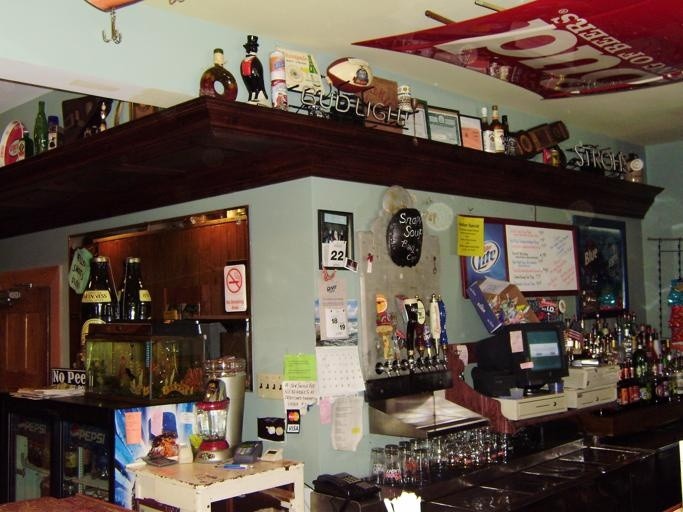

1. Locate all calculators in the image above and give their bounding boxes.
[142,455,177,467]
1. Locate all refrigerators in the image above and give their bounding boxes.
[8,391,202,512]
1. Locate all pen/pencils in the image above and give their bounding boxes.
[224,464,248,467]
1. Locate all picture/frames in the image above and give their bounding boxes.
[319,209,355,270]
[460,212,581,299]
[404,99,484,151]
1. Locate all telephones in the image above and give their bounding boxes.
[313,472,382,500]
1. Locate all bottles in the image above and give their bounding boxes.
[566,311,683,406]
[308,55,321,87]
[27,437,109,500]
[117,257,151,321]
[502,114,516,156]
[78,256,117,369]
[18,128,33,162]
[33,101,49,156]
[489,106,505,155]
[481,107,495,154]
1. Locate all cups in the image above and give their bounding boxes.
[368,427,511,486]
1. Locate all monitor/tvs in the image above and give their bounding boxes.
[490,321,569,398]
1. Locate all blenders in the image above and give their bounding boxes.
[195,400,231,465]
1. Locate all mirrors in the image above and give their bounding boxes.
[572,215,630,320]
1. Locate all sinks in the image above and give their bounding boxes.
[529,458,601,478]
[483,470,572,495]
[564,446,640,468]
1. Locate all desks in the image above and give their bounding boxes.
[134,458,308,512]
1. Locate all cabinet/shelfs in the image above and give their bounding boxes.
[449,344,683,456]
[0,205,252,512]
